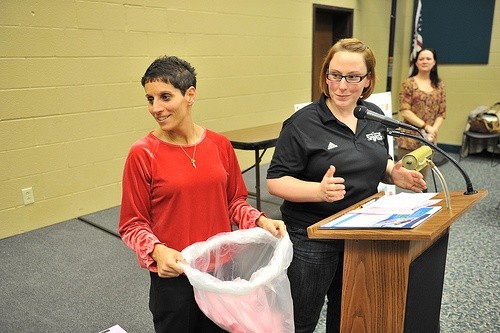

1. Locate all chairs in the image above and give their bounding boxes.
[463,113,500,167]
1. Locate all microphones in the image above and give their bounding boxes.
[353,105,418,131]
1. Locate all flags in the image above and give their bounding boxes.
[409,0,423,77]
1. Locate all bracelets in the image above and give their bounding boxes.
[423,122,427,128]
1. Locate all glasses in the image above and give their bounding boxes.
[326,72,369,83]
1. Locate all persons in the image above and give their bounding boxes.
[117,56,286,333]
[266,38,427,333]
[397,47,447,193]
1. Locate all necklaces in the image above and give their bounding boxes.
[164,123,196,168]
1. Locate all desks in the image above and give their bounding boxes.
[220,123,284,212]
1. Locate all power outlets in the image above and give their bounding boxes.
[22,186,35,205]
